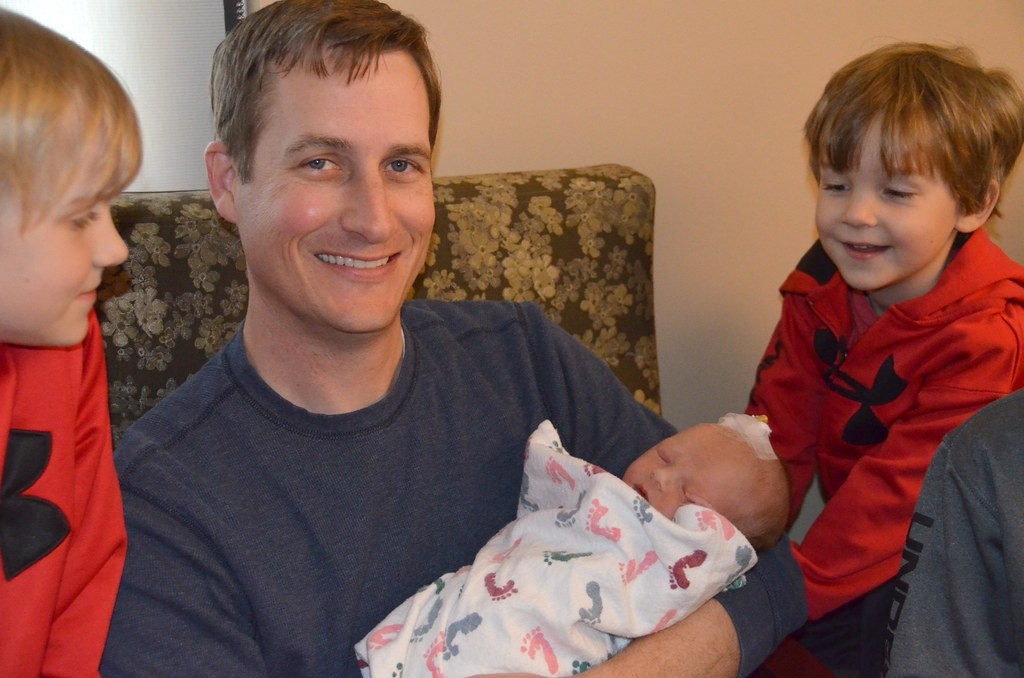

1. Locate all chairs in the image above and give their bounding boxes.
[91,161,665,449]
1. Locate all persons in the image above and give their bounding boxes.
[100,0,807,677]
[882,390,1024,677]
[0,4,144,677]
[741,38,1024,677]
[355,414,790,678]
